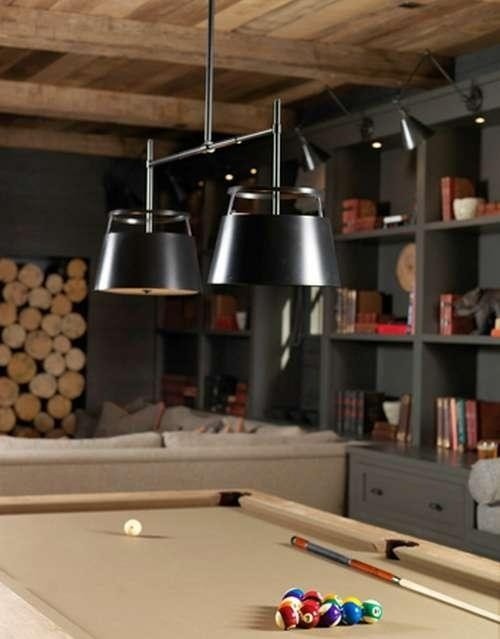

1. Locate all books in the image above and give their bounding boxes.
[334,389,413,442]
[440,293,476,335]
[209,293,246,332]
[342,197,380,233]
[163,371,247,416]
[436,396,500,453]
[441,174,475,221]
[334,286,382,334]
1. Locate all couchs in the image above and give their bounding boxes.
[0,404,348,518]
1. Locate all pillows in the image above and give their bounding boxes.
[73,396,245,439]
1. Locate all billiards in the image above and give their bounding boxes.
[124,520,141,536]
[275,588,383,630]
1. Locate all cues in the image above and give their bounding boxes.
[291,535,499,622]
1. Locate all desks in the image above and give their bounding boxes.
[0,487,500,639]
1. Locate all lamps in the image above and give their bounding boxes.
[391,48,484,154]
[93,1,343,297]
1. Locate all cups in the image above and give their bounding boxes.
[475,438,500,462]
